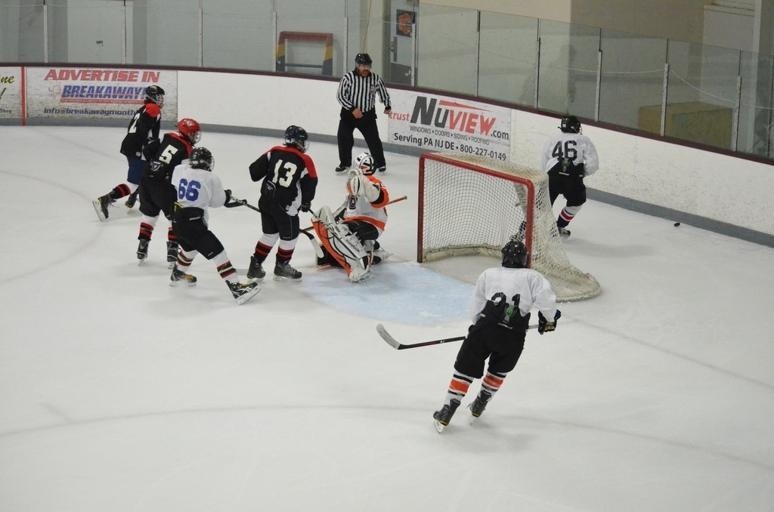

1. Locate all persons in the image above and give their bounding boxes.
[90,84,166,218]
[432,240,553,426]
[133,118,199,265]
[335,52,392,173]
[512,115,599,241]
[316,153,388,270]
[248,125,319,281]
[170,147,259,304]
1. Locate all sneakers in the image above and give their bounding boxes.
[433,398,461,426]
[136,243,148,259]
[166,250,180,262]
[273,254,303,279]
[98,194,117,219]
[469,389,492,417]
[170,263,197,283]
[379,163,387,171]
[246,256,266,279]
[226,279,257,299]
[335,162,348,171]
[549,227,571,237]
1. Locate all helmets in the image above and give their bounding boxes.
[189,146,215,172]
[174,117,201,146]
[353,152,378,175]
[283,125,310,153]
[559,113,582,134]
[143,84,165,109]
[355,53,373,64]
[501,240,529,269]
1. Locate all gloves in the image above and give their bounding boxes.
[538,309,562,335]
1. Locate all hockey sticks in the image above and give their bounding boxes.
[228,194,325,259]
[375,323,540,350]
[224,199,247,208]
